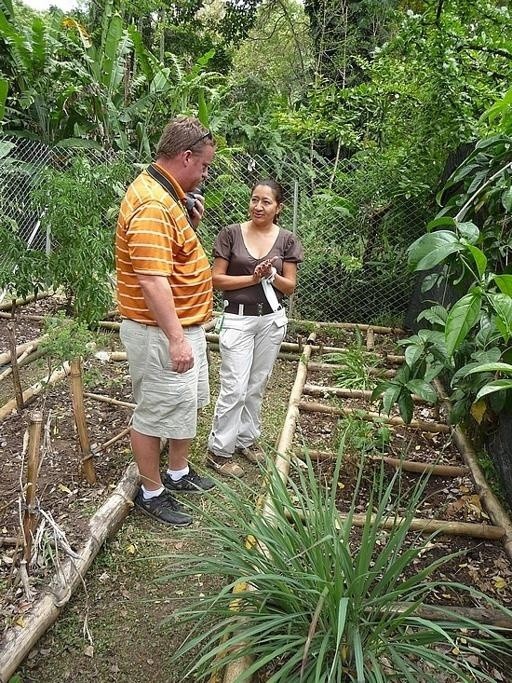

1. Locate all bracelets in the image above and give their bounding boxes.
[264,267,279,284]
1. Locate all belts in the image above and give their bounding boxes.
[225,299,282,316]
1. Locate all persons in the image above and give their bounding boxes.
[113,115,220,527]
[205,177,303,479]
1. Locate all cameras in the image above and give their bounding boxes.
[185,185,205,219]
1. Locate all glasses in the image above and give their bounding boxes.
[186,129,213,151]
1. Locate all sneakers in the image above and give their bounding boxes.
[133,487,193,526]
[163,464,216,493]
[239,443,266,464]
[205,449,245,478]
